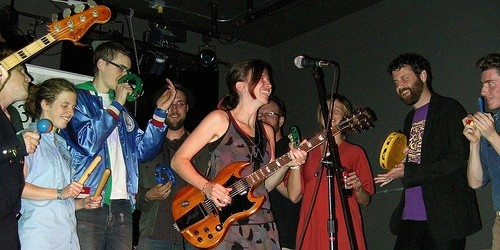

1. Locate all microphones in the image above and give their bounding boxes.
[294,55,330,68]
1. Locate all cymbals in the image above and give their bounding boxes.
[382,131,407,169]
[118,70,145,102]
[292,126,299,146]
[153,163,176,186]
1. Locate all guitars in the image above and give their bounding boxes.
[0,0,112,76]
[170,106,379,249]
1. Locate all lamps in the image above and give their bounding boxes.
[131,18,179,96]
[195,30,219,71]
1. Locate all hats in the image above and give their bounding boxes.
[153,63,196,113]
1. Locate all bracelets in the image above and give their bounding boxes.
[290,166,301,170]
[203,183,207,192]
[81,200,84,208]
[57,189,62,200]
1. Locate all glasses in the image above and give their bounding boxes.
[258,111,281,118]
[169,101,187,109]
[103,58,131,75]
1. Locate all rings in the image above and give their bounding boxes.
[389,178,391,180]
[164,190,168,192]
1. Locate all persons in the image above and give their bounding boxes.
[283,93,375,250]
[170,60,306,250]
[256,96,302,250]
[0,42,210,250]
[462,53,500,250]
[372,52,483,250]
